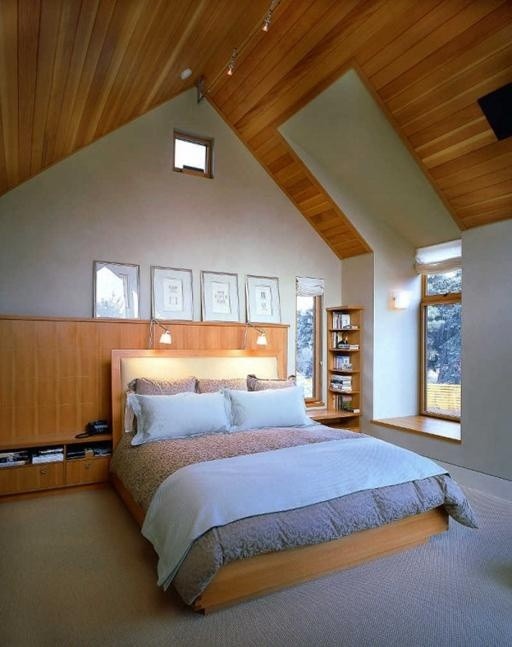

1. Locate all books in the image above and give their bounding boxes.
[330,311,360,413]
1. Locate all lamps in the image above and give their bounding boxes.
[238,323,269,352]
[142,318,174,351]
[196,0,280,105]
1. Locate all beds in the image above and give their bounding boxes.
[109,348,482,614]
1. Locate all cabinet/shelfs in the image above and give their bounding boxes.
[326,303,365,437]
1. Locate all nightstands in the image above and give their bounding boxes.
[305,407,362,433]
[0,432,112,499]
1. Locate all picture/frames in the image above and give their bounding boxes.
[148,264,195,323]
[198,268,241,323]
[246,270,280,323]
[90,260,141,321]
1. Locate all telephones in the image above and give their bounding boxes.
[87,419,110,436]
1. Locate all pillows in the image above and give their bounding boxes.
[127,376,197,434]
[250,373,296,391]
[128,391,231,445]
[222,388,311,433]
[191,376,247,392]
